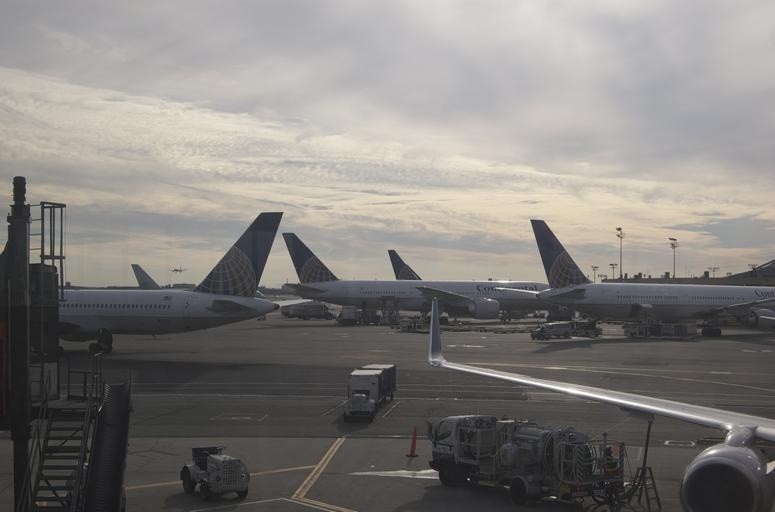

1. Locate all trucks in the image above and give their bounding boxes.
[425,414,627,509]
[180,444,251,500]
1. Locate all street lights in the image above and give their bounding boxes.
[591,225,678,280]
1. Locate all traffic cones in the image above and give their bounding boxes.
[405,426,419,457]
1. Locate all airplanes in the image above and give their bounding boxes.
[281,231,549,322]
[57,210,284,355]
[530,218,775,338]
